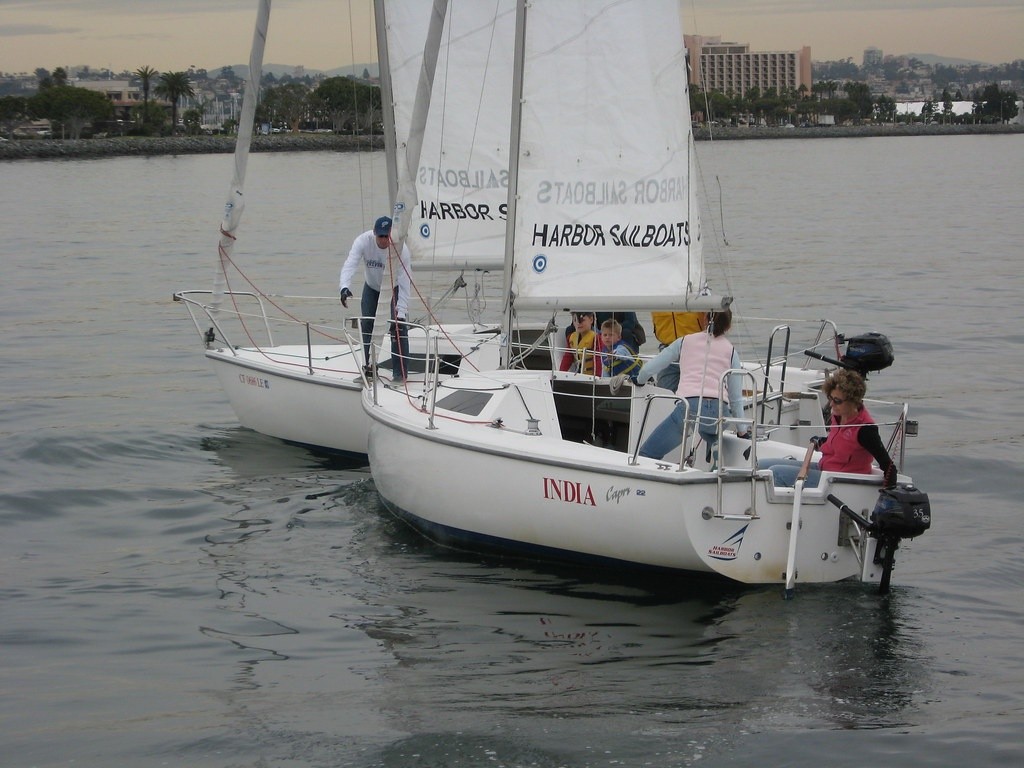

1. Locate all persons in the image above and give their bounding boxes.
[650,311,707,393]
[560,312,656,382]
[756,369,898,488]
[629,308,749,460]
[340,215,412,386]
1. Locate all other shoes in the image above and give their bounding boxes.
[353,371,378,383]
[388,378,406,387]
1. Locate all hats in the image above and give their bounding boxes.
[374,216,392,235]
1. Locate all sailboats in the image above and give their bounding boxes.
[168,0,895,460]
[336,0,933,589]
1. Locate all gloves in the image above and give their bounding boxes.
[632,376,645,387]
[737,433,749,439]
[341,288,353,308]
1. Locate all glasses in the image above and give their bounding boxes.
[379,234,388,239]
[827,395,847,405]
